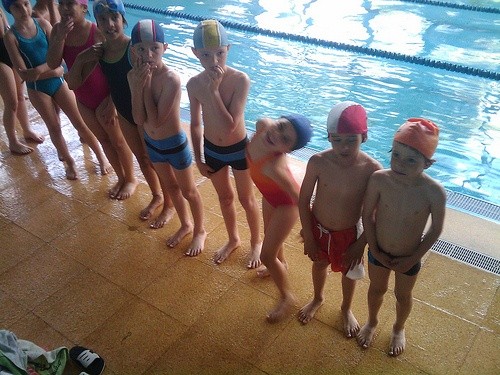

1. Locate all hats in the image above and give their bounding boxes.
[393,116,439,160]
[193,19,231,52]
[92,0,126,20]
[130,18,165,46]
[326,100,369,135]
[281,113,314,151]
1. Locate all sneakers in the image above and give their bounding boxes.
[69,345,106,375]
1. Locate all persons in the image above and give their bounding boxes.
[185,19,263,269]
[354,117,447,356]
[0,0,208,257]
[298,100,384,338]
[245,113,312,324]
